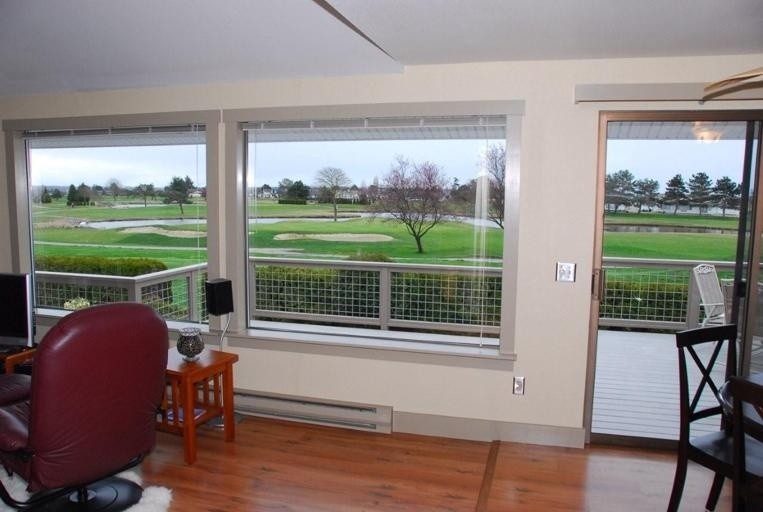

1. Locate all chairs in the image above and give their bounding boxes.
[666,323,763,511]
[692,264,726,327]
[0,302,168,511]
[732,378,763,512]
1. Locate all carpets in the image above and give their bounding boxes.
[0,464,172,511]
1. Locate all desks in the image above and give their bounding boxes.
[717,373,763,511]
[154,344,238,465]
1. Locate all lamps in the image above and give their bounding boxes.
[204,277,241,427]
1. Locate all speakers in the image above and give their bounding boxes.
[205,279,233,315]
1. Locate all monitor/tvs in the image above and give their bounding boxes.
[0,272,33,353]
